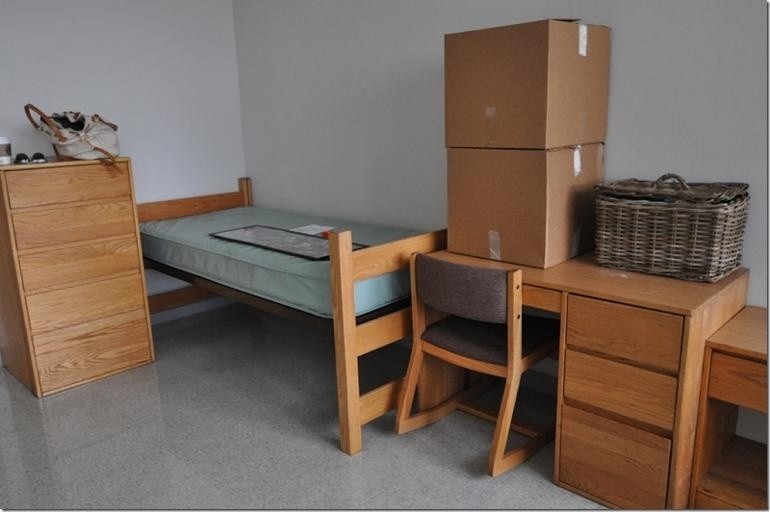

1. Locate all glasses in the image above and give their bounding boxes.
[15,153,46,163]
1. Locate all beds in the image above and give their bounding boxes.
[137,178,447,456]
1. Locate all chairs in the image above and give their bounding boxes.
[395,253,555,477]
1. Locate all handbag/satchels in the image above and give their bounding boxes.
[24,104,119,160]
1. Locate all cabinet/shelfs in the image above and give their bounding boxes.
[688,306,768,510]
[416,249,749,510]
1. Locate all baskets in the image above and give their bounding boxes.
[593,177,750,283]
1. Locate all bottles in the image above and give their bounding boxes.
[0,137,12,165]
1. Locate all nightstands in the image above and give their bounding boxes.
[0,157,155,399]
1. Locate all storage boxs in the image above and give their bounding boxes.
[444,18,611,150]
[447,143,606,269]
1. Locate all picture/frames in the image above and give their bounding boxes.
[208,224,370,260]
